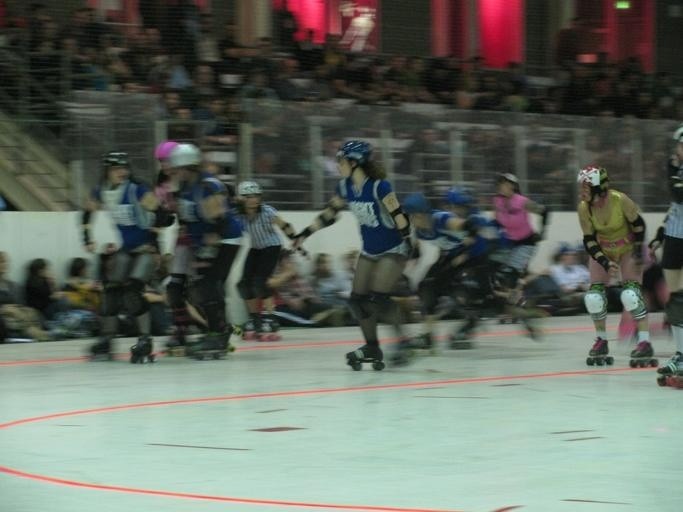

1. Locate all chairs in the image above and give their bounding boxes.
[74,131,682,213]
[6,37,683,131]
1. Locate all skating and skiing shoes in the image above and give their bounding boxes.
[165,328,236,359]
[586,339,615,366]
[657,348,683,390]
[392,301,547,364]
[255,314,282,342]
[342,342,386,372]
[239,313,259,341]
[87,340,114,363]
[129,335,157,365]
[629,340,658,369]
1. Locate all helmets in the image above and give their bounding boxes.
[443,189,469,207]
[498,172,520,189]
[403,193,432,216]
[166,143,204,167]
[673,126,682,143]
[154,140,179,162]
[575,164,611,193]
[236,182,263,197]
[336,139,374,165]
[99,148,133,170]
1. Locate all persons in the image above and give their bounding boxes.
[491,171,549,317]
[439,184,529,339]
[291,139,422,364]
[148,139,199,346]
[404,194,541,351]
[77,149,156,358]
[576,166,656,358]
[162,145,243,357]
[235,179,310,331]
[646,126,682,373]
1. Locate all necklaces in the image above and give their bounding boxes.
[353,175,369,194]
